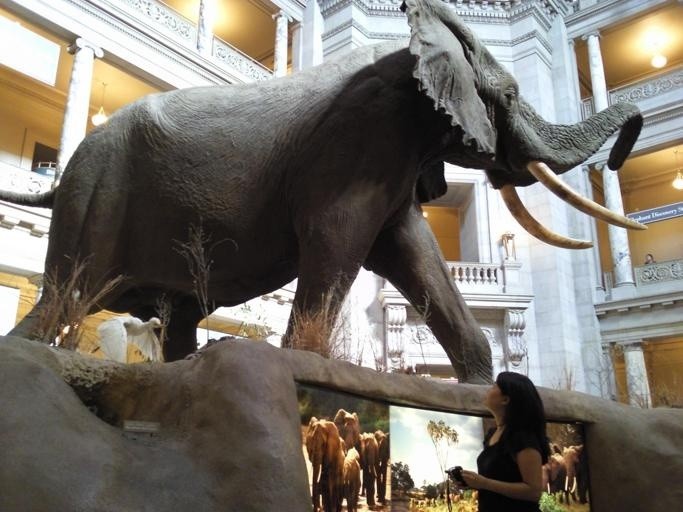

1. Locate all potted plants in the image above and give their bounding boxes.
[391,462,414,512]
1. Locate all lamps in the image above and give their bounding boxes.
[650,48,668,69]
[92,83,109,126]
[673,150,683,189]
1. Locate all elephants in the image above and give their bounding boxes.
[541,443,588,504]
[0,0,647,385]
[305,409,389,512]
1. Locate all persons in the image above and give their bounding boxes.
[642,253,660,282]
[445,370,549,512]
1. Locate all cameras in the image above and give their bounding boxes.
[449,467,464,481]
